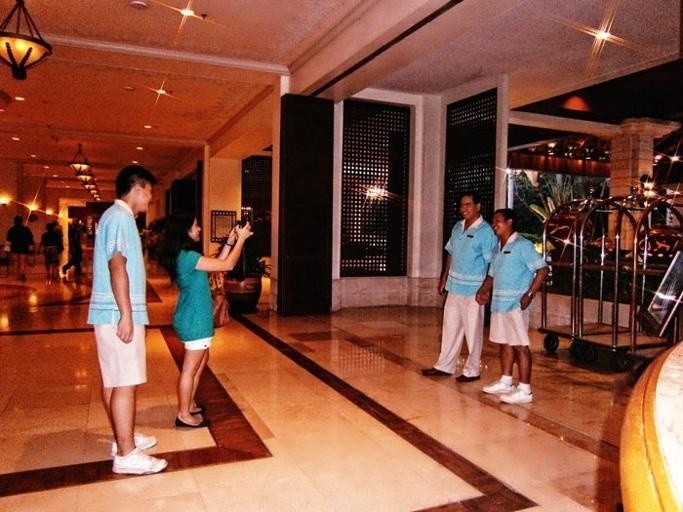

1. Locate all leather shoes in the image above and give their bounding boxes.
[175,417,209,427]
[423,369,451,376]
[190,406,204,414]
[456,375,480,382]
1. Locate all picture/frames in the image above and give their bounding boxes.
[210,210,237,243]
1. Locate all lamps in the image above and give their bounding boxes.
[0,0,52,80]
[69,142,103,202]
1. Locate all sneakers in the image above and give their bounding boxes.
[500,389,533,403]
[112,448,168,474]
[482,382,512,393]
[111,433,156,457]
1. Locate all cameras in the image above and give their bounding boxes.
[238,216,249,227]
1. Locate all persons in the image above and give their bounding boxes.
[85,165,168,475]
[62,217,84,276]
[475,209,550,406]
[161,211,255,427]
[37,220,65,280]
[6,216,35,281]
[421,190,498,382]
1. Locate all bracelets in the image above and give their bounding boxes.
[525,291,534,300]
[224,242,234,246]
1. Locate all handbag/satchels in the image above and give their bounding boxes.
[212,288,230,326]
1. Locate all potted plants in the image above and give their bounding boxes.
[215,214,272,314]
